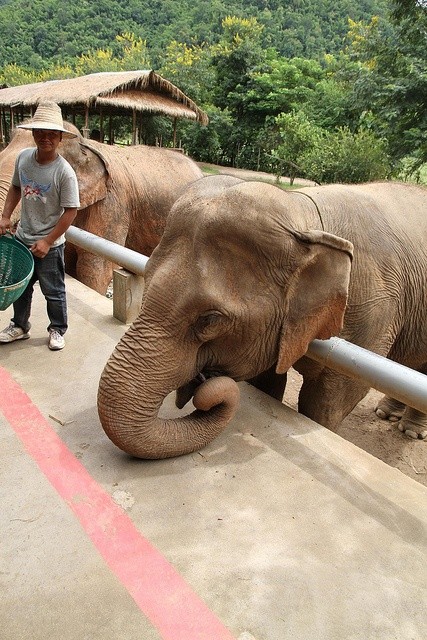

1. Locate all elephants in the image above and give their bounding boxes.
[98,171,427,460]
[0,120,203,297]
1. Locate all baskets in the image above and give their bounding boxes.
[1,229,35,311]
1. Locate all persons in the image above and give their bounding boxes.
[0,96,80,350]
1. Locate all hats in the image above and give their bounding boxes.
[17,100,78,139]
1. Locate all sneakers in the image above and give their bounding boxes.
[1,321,31,343]
[49,329,66,351]
